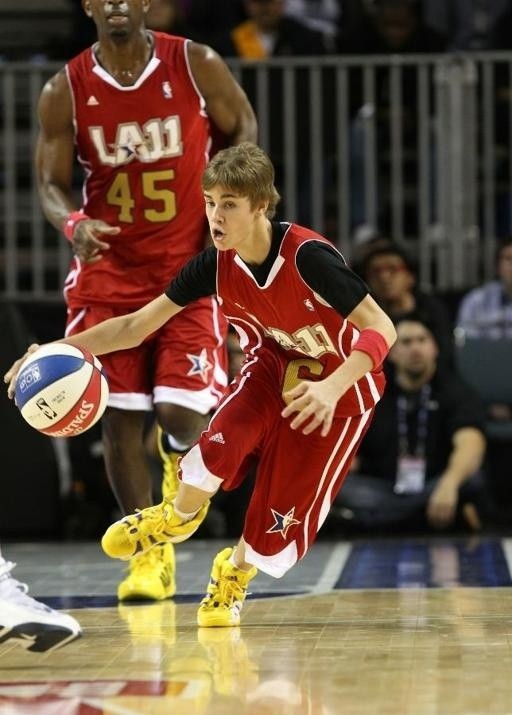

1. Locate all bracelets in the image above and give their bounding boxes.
[351,328,390,371]
[64,212,89,241]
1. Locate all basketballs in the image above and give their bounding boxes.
[14,342,109,438]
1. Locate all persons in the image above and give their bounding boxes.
[0,142,397,627]
[34,0,259,601]
[335,240,512,534]
[0,549,83,662]
[0,0,460,57]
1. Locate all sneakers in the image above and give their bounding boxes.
[0,555,84,655]
[102,425,211,602]
[195,546,258,627]
